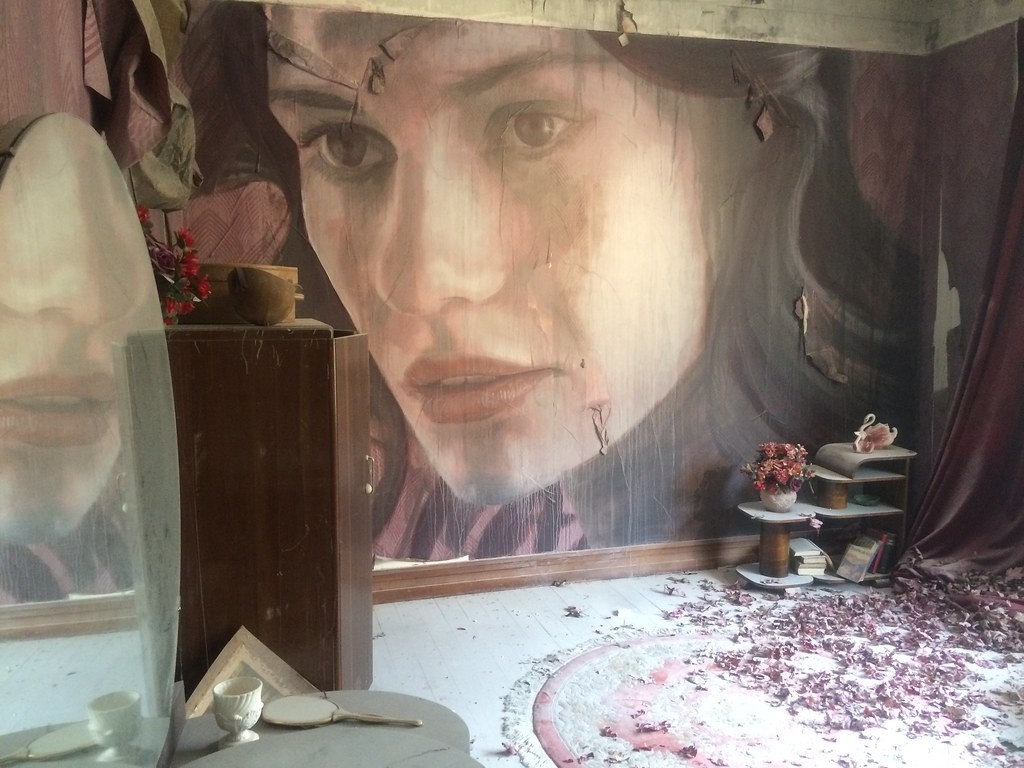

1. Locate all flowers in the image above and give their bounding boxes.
[737,441,816,495]
[136,205,212,326]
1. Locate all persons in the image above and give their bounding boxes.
[0,118,165,605]
[183,0,914,547]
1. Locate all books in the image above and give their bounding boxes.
[790,538,835,576]
[836,527,897,582]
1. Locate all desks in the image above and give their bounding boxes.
[0,690,485,768]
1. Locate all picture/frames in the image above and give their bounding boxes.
[186,625,322,718]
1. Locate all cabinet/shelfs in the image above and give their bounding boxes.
[735,501,817,590]
[797,444,916,584]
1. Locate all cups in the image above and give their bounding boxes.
[86,691,144,764]
[213,676,264,749]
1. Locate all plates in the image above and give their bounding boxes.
[853,494,881,505]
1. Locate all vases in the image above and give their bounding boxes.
[758,487,796,513]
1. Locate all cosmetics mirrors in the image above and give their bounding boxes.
[1,722,101,765]
[262,696,423,728]
